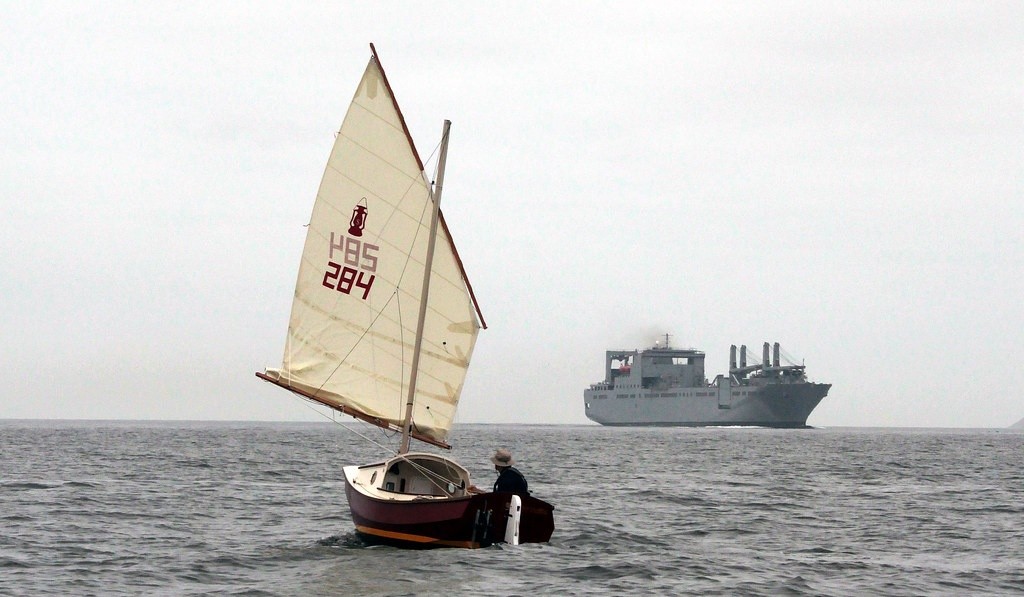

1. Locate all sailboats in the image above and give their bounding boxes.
[255,43,556,550]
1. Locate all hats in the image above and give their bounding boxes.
[491,448,514,467]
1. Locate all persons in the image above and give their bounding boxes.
[489,449,531,497]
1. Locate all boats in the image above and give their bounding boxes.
[583,333,832,428]
